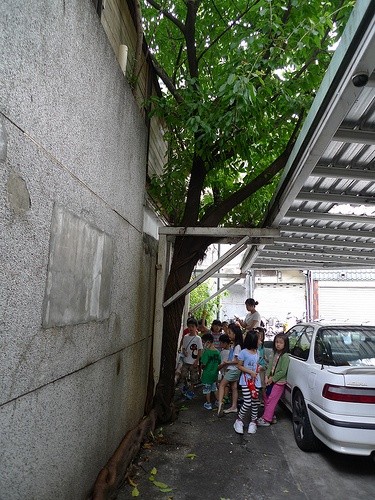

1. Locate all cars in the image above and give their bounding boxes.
[264,321,375,458]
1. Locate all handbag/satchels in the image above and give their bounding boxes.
[264,371,274,395]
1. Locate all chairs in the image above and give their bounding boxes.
[315,340,335,366]
[360,338,375,360]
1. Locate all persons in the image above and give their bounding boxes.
[217,323,243,416]
[233,330,261,435]
[200,334,222,410]
[234,298,261,339]
[256,333,290,427]
[178,317,269,406]
[181,318,203,392]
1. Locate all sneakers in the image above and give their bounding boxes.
[204,402,212,409]
[248,421,257,433]
[232,419,244,434]
[215,401,219,407]
[256,418,270,426]
[271,416,277,423]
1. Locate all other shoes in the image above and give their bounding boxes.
[182,385,188,394]
[185,389,195,397]
[218,403,224,417]
[223,408,237,413]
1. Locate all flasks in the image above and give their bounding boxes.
[248,379,258,398]
